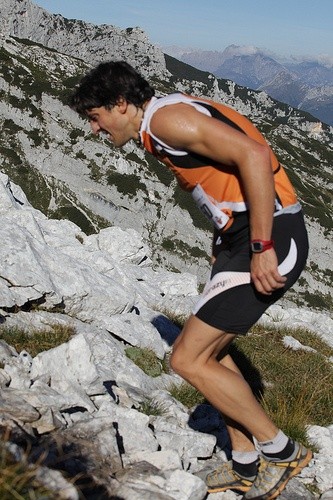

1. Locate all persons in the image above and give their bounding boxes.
[76,60,312,500]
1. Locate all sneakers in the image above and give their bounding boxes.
[243,437,312,500]
[204,458,260,493]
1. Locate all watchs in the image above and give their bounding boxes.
[250,239,275,254]
